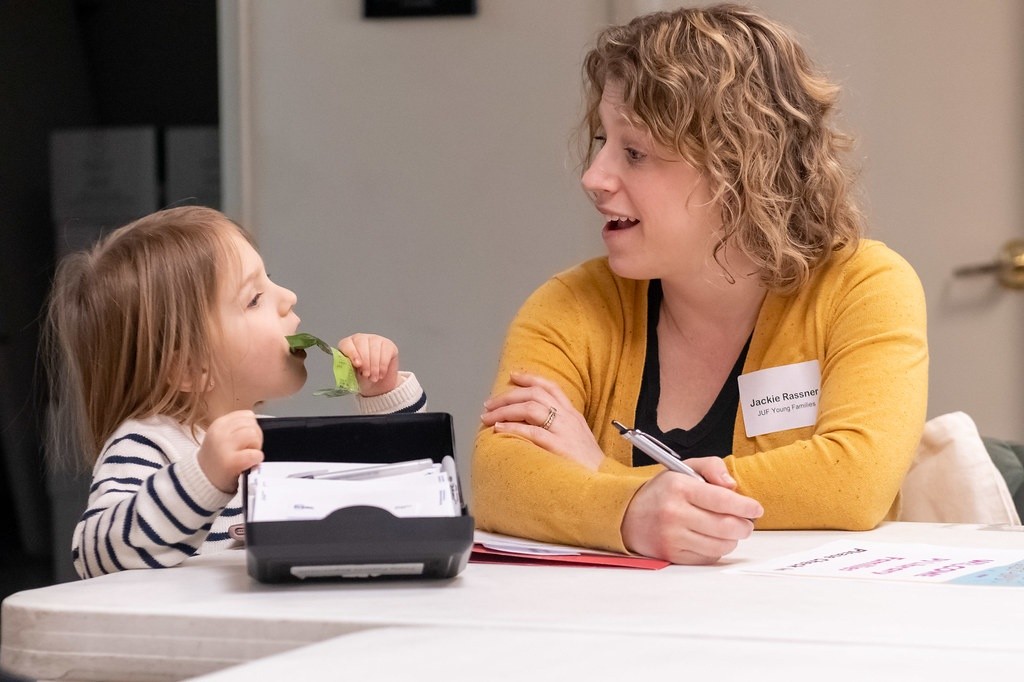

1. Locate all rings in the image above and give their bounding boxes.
[540,407,557,430]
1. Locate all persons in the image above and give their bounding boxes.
[470,0,929,566]
[47,206,429,581]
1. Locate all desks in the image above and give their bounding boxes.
[0,521,1024,682]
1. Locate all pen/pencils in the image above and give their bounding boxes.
[610,417,707,484]
[292,456,434,480]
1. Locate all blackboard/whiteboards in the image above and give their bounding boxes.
[218,0,1024,510]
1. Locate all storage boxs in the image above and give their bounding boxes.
[243,411,477,584]
